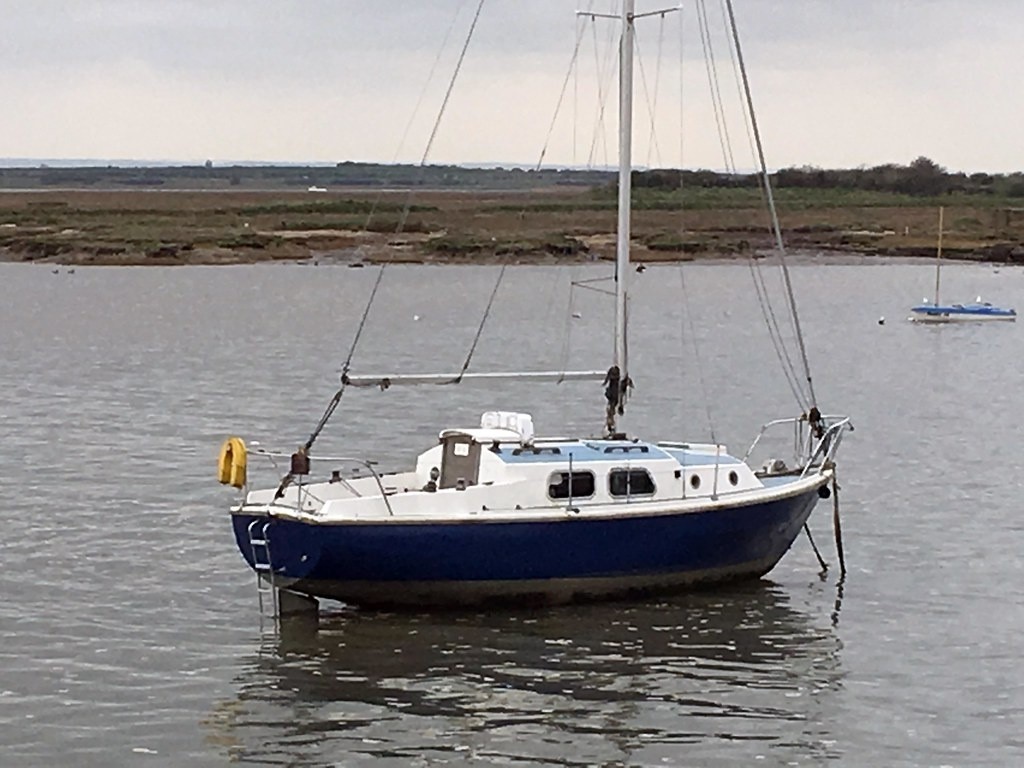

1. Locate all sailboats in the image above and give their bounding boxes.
[909,206,1017,322]
[215,1,854,611]
